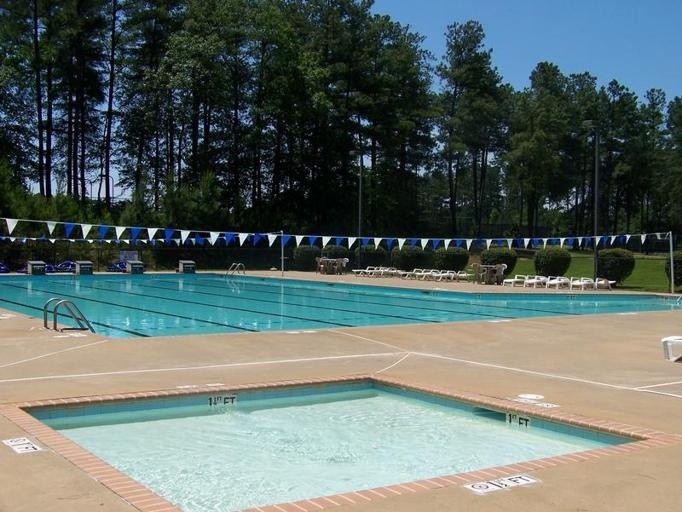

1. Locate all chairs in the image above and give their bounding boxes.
[352,264,620,293]
[314,256,350,276]
[660,334,682,363]
